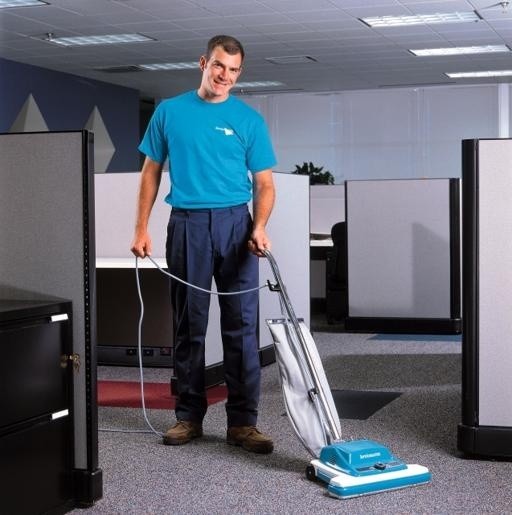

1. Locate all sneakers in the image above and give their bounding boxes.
[162,419,202,446]
[226,426,273,454]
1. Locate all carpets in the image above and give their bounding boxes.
[367,332,462,343]
[94,379,228,412]
[282,388,403,422]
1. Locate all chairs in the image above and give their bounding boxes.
[328,221,347,322]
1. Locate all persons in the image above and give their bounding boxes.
[129,33,279,456]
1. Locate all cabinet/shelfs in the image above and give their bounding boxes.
[0,284,79,514]
